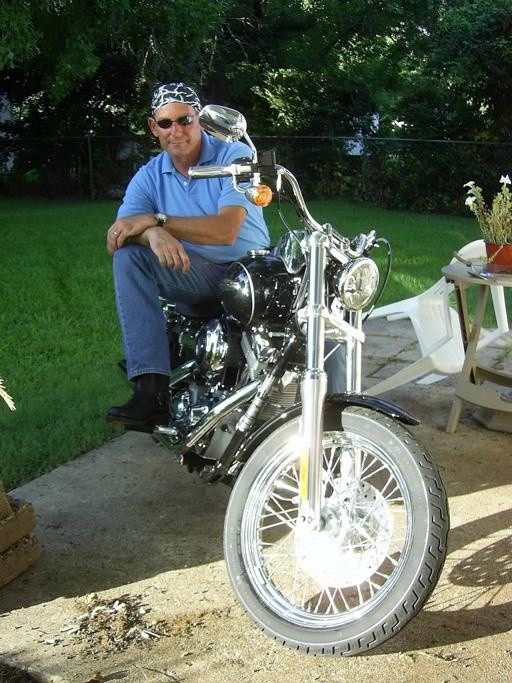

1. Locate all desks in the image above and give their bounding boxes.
[440,261,512,439]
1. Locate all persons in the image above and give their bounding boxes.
[103,82,273,427]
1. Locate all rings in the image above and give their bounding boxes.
[113,231,120,237]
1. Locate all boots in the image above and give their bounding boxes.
[105,373,169,426]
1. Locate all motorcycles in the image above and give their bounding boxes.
[116,103,450,659]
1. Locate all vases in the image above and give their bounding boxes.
[485,243,512,275]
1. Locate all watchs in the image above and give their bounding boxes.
[155,213,168,228]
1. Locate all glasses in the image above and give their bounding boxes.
[153,112,197,129]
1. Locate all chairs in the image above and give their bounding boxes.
[357,239,509,399]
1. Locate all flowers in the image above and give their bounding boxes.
[461,170,511,263]
